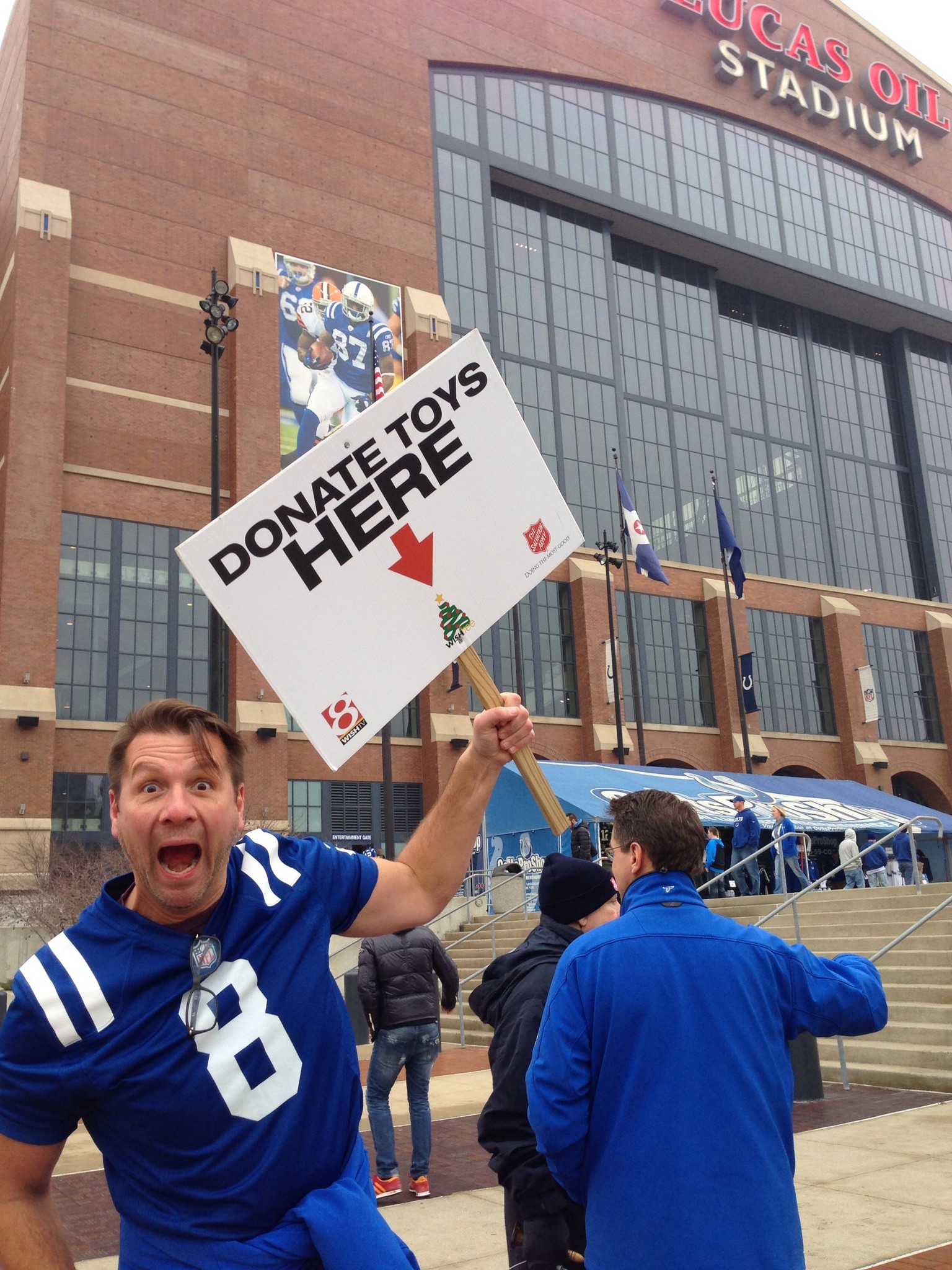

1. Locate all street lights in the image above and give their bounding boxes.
[594,529,625,764]
[198,265,239,717]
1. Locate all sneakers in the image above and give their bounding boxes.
[408,1172,430,1197]
[371,1175,403,1199]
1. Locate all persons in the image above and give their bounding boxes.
[358,924,459,1198]
[706,795,932,897]
[363,844,377,858]
[0,692,535,1270]
[276,256,402,459]
[566,813,594,862]
[469,852,621,1270]
[377,849,385,859]
[525,790,888,1270]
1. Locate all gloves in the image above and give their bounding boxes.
[522,1210,573,1270]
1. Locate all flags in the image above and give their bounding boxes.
[615,469,669,585]
[712,487,746,599]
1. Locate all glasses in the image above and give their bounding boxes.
[186,934,221,1039]
[604,841,645,862]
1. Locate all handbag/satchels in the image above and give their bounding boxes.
[579,843,597,858]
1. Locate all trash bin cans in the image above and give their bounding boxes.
[492,863,525,914]
[344,969,370,1045]
[786,1029,825,1103]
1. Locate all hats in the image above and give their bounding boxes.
[868,832,876,839]
[538,852,619,925]
[729,795,745,802]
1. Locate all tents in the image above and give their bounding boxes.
[464,758,952,914]
